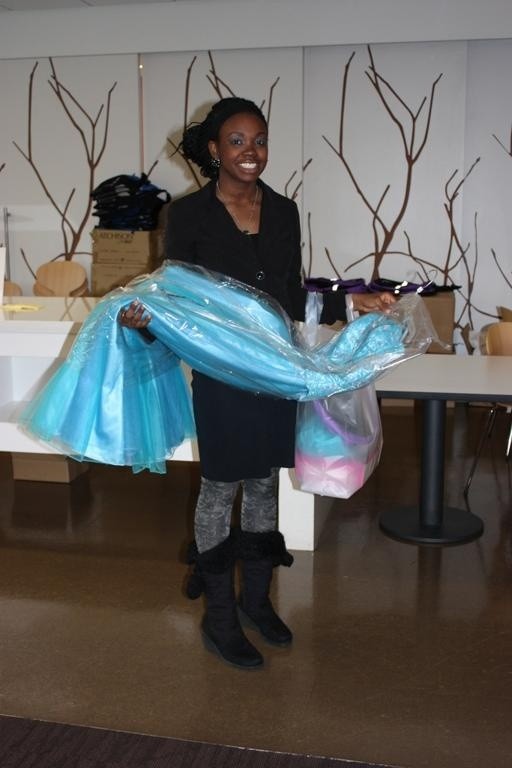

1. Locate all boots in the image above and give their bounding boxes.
[236,530,294,648]
[184,526,264,669]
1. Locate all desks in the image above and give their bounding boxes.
[373,353,512,547]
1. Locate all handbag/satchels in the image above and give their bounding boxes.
[294,291,384,500]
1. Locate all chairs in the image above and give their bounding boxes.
[463,306,512,499]
[4,260,89,297]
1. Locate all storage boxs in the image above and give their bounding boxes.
[358,289,456,355]
[89,202,171,297]
[11,452,90,484]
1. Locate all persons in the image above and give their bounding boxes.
[117,97,397,669]
